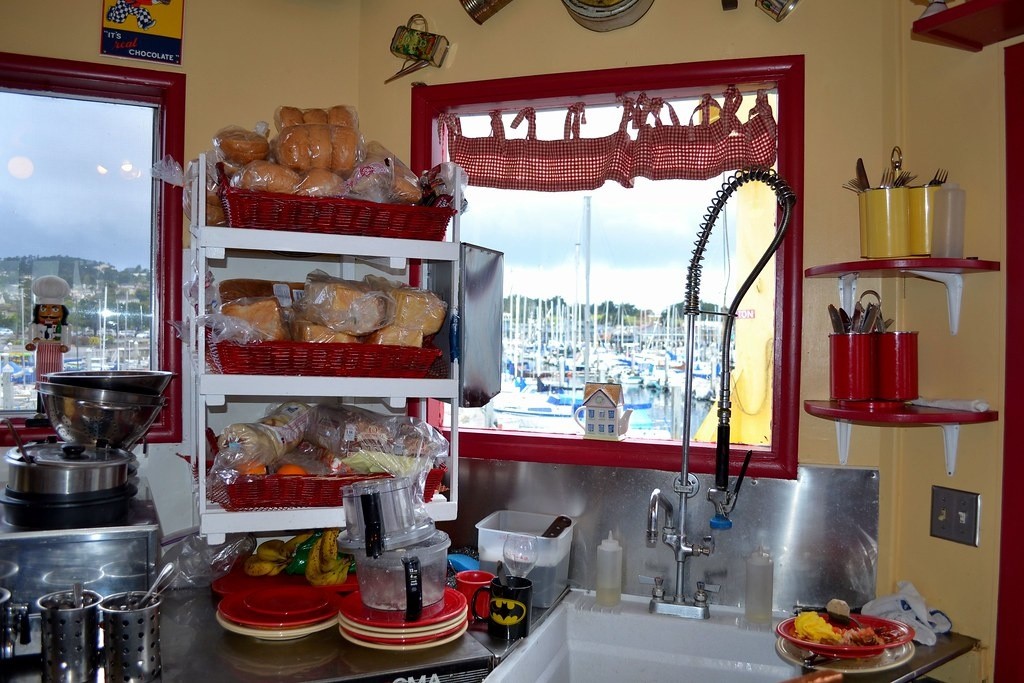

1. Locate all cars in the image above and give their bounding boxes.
[136,331,149,338]
[0,329,13,335]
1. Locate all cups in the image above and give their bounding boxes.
[471,576,533,642]
[455,570,495,620]
[503,533,538,578]
[574,379,633,441]
[856,185,942,258]
[877,331,920,401]
[828,332,877,401]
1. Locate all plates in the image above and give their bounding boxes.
[776,636,915,673]
[243,590,329,615]
[776,614,915,658]
[339,586,469,650]
[211,566,356,593]
[218,593,339,630]
[216,610,340,640]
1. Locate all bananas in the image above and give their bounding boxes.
[243,530,314,576]
[305,527,352,585]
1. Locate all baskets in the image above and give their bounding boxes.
[215,162,458,242]
[197,425,448,510]
[202,323,443,380]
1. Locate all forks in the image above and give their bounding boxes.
[842,168,949,194]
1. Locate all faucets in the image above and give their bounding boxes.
[645,488,715,620]
[0,601,32,659]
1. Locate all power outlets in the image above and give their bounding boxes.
[931,485,980,547]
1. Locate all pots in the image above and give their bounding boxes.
[2,436,137,495]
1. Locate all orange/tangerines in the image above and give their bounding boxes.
[276,464,306,474]
[233,461,267,474]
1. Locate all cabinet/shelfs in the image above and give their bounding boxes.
[191,153,460,546]
[803,257,1001,477]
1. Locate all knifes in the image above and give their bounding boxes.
[828,303,844,333]
[852,301,861,332]
[840,307,851,333]
[856,157,870,191]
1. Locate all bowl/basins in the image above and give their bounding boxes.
[336,477,417,559]
[353,530,451,622]
[36,371,178,406]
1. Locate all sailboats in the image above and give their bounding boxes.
[0,282,151,384]
[503,293,735,412]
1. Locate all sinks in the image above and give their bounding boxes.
[486,609,795,683]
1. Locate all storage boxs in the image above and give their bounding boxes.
[475,508,579,609]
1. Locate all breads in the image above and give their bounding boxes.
[216,274,449,348]
[218,402,444,476]
[184,106,423,228]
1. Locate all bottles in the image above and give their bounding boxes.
[931,184,966,258]
[745,545,773,626]
[595,530,622,606]
